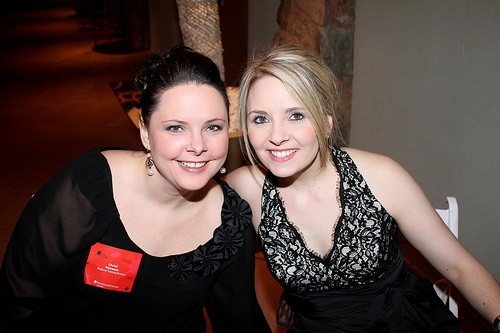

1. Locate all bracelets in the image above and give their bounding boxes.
[493,315,500,329]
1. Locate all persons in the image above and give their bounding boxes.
[0,46,273,333]
[219,42,500,333]
